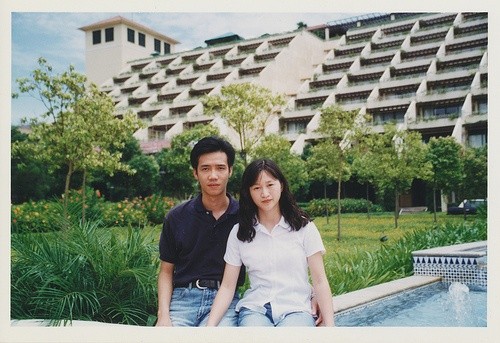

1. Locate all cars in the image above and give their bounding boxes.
[462,199,487,216]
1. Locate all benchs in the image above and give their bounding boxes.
[398,207,428,215]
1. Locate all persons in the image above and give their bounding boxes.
[154,135,325,327]
[205,157,337,328]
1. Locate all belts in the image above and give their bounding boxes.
[173,278,223,290]
